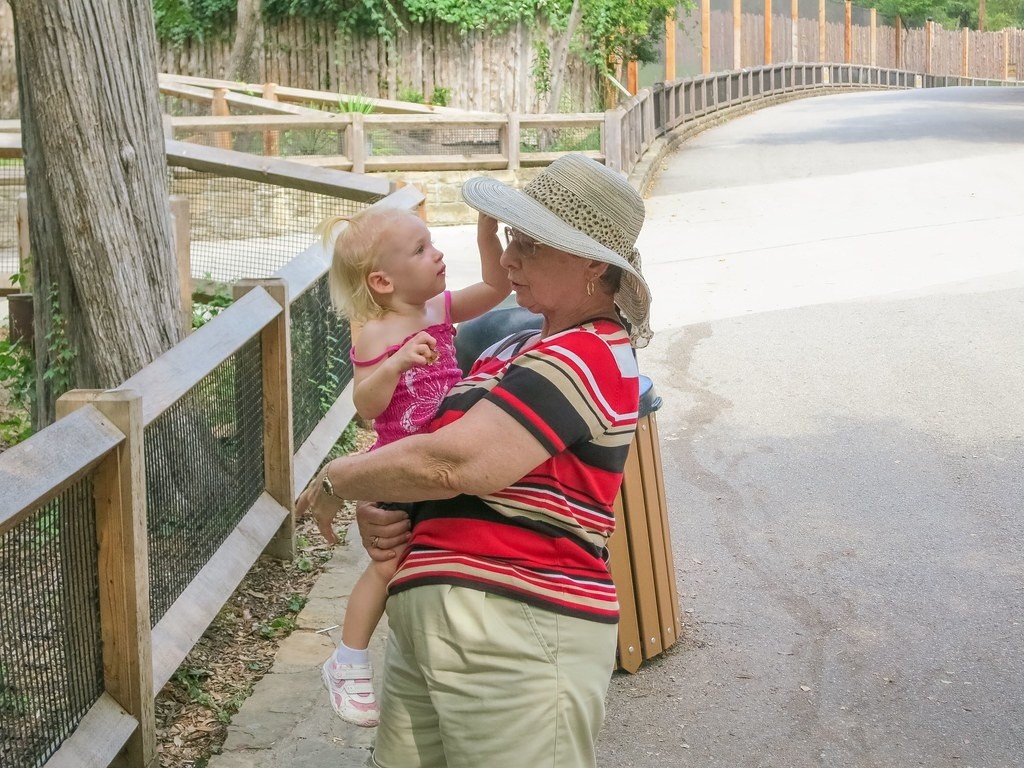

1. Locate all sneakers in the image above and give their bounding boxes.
[321,654,380,727]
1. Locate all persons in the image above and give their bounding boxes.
[295,151,655,768]
[318,201,511,727]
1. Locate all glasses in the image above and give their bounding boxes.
[505,226,547,257]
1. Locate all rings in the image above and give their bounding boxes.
[370,536,379,549]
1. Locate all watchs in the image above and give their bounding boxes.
[321,459,344,503]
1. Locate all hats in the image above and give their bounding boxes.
[460,154,653,351]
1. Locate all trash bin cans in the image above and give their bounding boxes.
[603,375,681,674]
